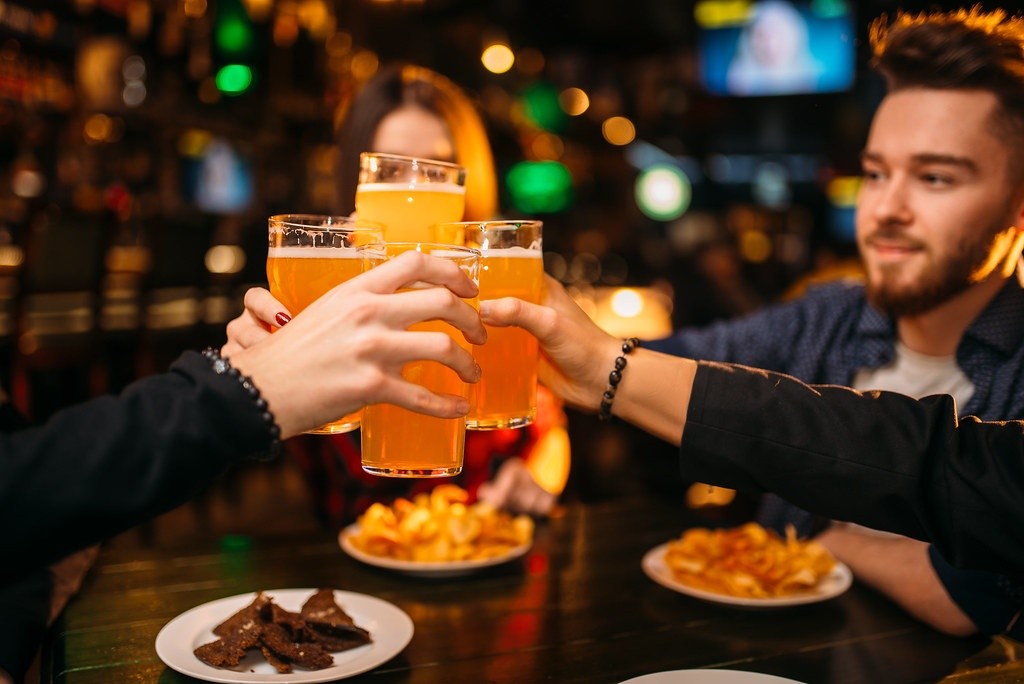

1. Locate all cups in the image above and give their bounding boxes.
[425,219,542,433]
[266,214,384,437]
[352,242,479,479]
[352,153,470,248]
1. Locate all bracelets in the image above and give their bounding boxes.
[597,336,640,422]
[203,346,280,444]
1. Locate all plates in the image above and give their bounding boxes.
[333,519,533,578]
[619,668,805,684]
[641,541,853,606]
[156,587,414,684]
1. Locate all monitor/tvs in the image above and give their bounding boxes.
[687,0,867,108]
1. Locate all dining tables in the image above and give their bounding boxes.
[45,486,994,684]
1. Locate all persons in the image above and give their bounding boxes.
[474,11,1024,639]
[0,65,569,664]
